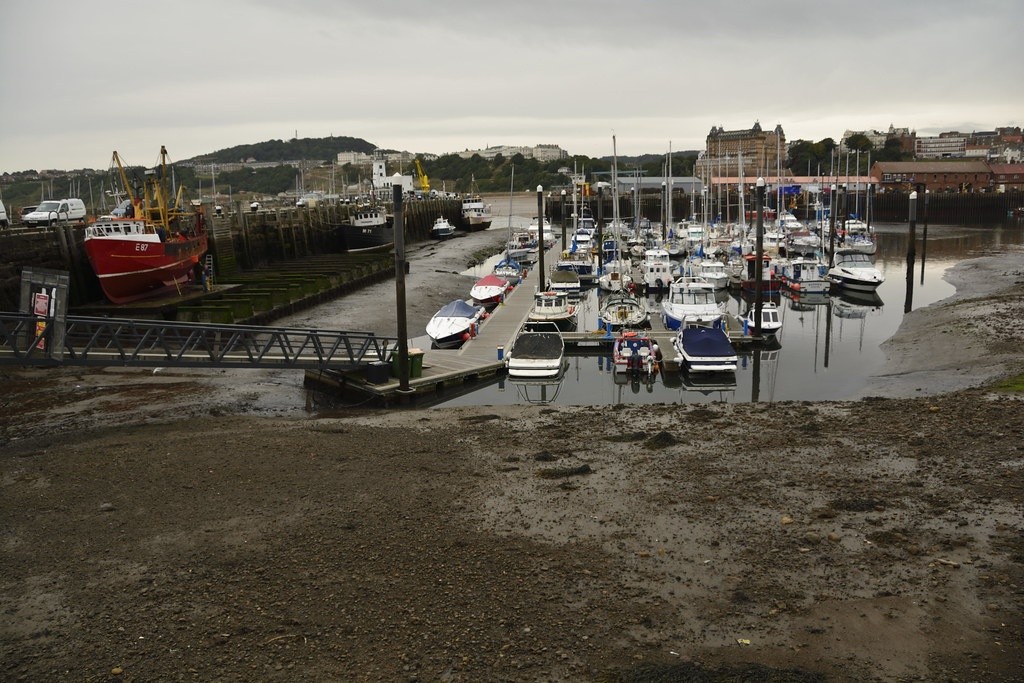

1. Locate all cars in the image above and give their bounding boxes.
[0,200,9,231]
[110,200,134,219]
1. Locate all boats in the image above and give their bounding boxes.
[332,198,407,254]
[528,225,555,247]
[426,299,486,349]
[529,277,782,335]
[775,211,804,231]
[613,331,659,373]
[493,259,522,284]
[546,248,886,304]
[431,216,456,237]
[470,274,511,307]
[458,174,492,232]
[505,321,565,380]
[670,312,737,374]
[84,145,209,302]
[504,232,539,264]
[568,217,878,261]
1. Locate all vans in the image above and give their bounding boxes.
[20,198,86,228]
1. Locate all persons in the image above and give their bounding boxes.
[156,223,165,242]
[199,262,210,291]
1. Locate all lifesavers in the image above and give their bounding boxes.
[622,332,638,337]
